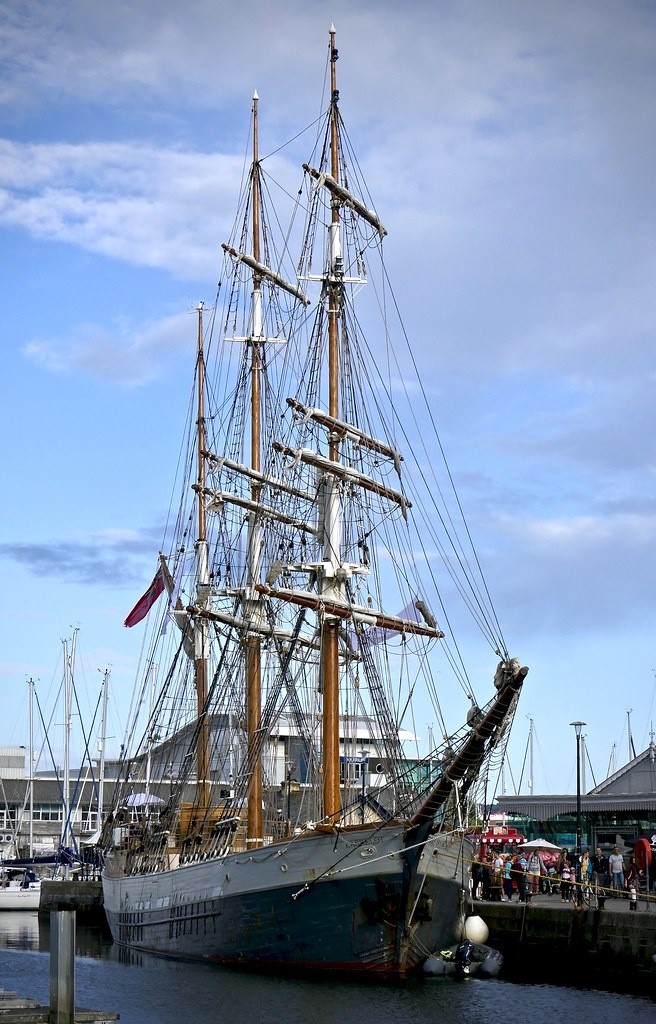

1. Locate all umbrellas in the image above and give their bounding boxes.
[516,837,562,850]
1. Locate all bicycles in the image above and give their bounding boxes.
[544,870,558,897]
[570,870,606,908]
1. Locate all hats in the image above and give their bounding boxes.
[571,867,575,870]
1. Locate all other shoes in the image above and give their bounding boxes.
[566,899,569,903]
[473,897,480,901]
[561,899,565,902]
[509,899,513,902]
[530,890,532,893]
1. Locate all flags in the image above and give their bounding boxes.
[123,564,165,628]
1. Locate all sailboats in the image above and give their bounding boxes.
[0,625,109,913]
[98,23,530,985]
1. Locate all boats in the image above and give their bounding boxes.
[420,916,504,982]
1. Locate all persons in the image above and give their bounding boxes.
[472,847,609,905]
[608,846,629,901]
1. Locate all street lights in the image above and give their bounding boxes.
[568,721,586,878]
